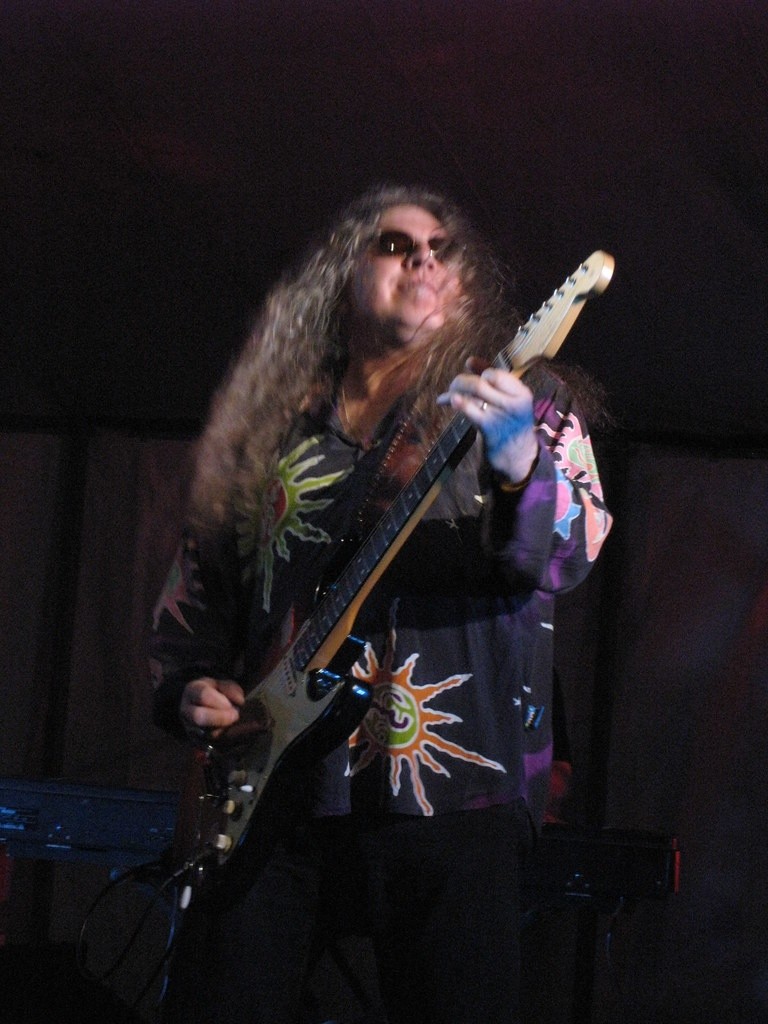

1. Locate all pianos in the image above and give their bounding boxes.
[0,777,686,903]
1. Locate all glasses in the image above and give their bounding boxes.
[379,231,458,263]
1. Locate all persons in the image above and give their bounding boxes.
[147,189,613,1024]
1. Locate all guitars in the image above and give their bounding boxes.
[166,246,616,914]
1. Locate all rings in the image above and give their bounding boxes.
[480,401,487,412]
